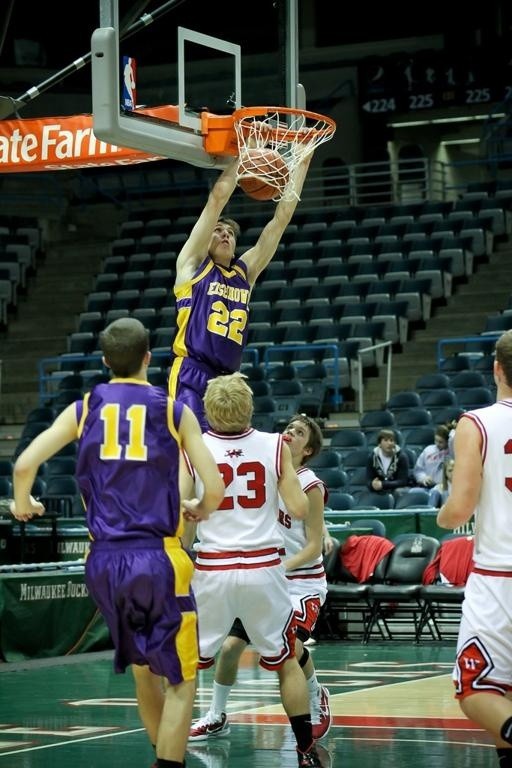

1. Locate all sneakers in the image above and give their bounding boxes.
[295,741,324,768]
[187,711,232,741]
[311,686,332,741]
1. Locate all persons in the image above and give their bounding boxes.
[428,459,456,510]
[412,418,457,488]
[365,428,408,490]
[176,374,332,768]
[304,520,333,646]
[9,317,226,767]
[437,324,512,768]
[165,119,318,553]
[188,414,333,743]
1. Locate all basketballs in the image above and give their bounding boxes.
[238,149,288,202]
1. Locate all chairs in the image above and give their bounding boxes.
[0,188,512,641]
